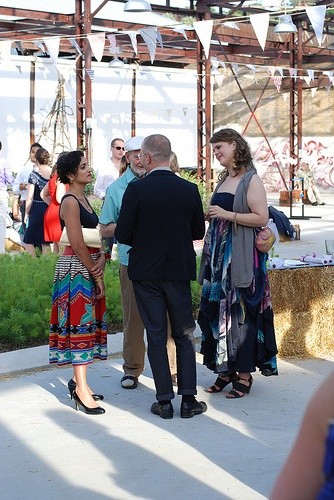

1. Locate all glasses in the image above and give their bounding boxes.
[137,153,152,160]
[114,147,124,151]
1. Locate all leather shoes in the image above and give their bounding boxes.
[181,399,207,418]
[151,400,173,419]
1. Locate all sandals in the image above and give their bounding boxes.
[171,374,178,386]
[121,373,138,388]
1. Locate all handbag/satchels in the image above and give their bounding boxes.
[255,226,276,254]
[59,226,102,249]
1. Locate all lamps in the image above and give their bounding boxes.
[109,53,124,68]
[123,0,153,12]
[274,0,298,33]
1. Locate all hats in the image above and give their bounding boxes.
[125,135,145,151]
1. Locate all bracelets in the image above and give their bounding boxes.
[93,274,102,284]
[99,249,106,254]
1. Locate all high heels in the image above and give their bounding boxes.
[72,390,105,414]
[226,374,252,398]
[207,371,237,393]
[68,379,104,400]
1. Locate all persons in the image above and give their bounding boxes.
[114,135,207,419]
[12,143,69,258]
[296,162,326,206]
[93,138,181,264]
[49,151,108,415]
[197,129,278,399]
[99,136,177,389]
[269,367,334,500]
[0,140,14,254]
